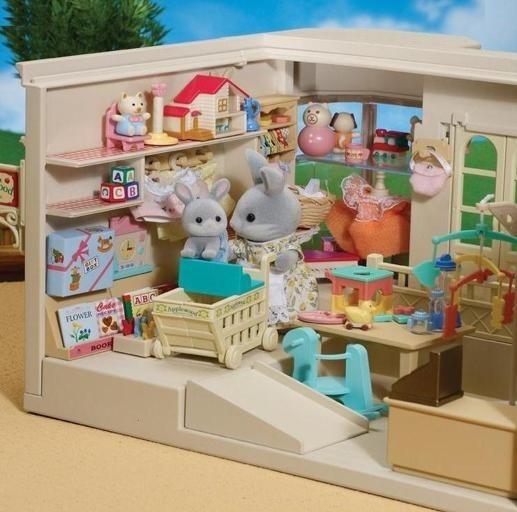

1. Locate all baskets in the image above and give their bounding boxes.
[288,184,336,229]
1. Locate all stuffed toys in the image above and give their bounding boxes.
[174,179,233,264]
[230,149,319,323]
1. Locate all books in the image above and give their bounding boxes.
[58,287,158,348]
